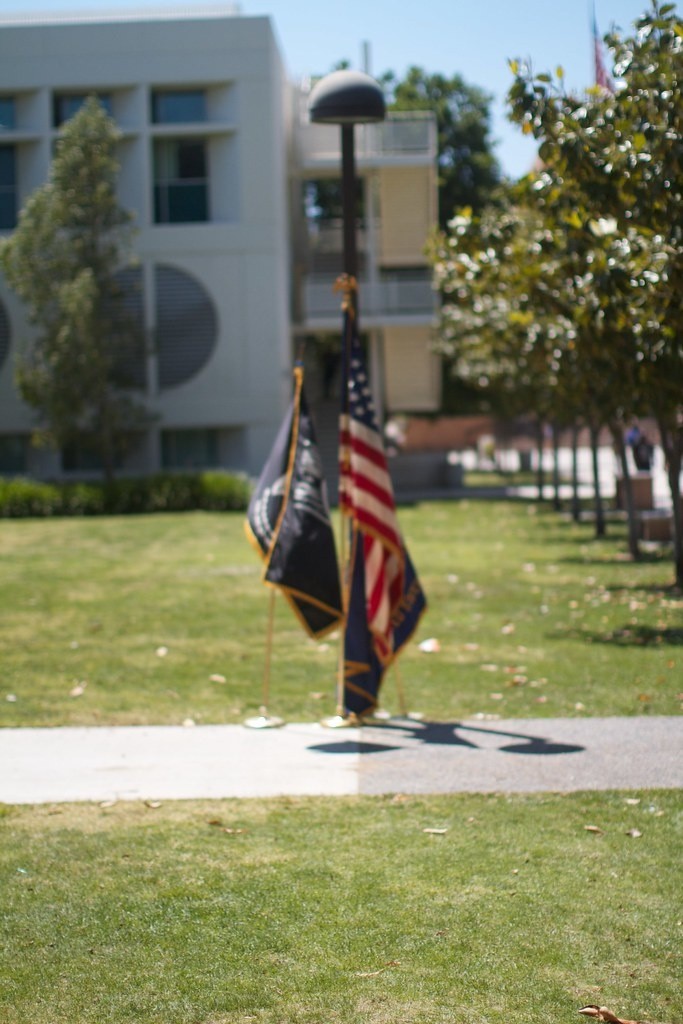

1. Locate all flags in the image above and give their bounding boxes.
[242,361,343,641]
[591,21,615,96]
[334,300,427,726]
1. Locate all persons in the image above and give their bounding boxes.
[627,425,656,473]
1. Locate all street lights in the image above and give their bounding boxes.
[305,68,388,368]
